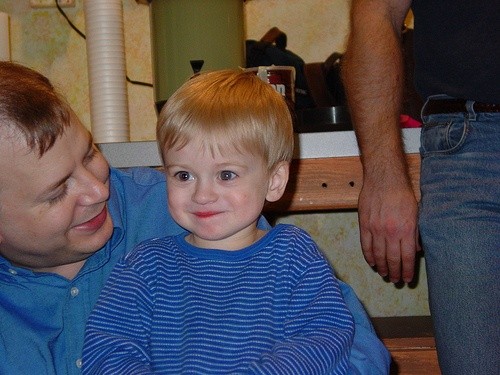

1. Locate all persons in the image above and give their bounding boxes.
[80,69,356,375]
[341,0,500,375]
[0,63,392,375]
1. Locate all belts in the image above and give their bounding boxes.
[418,99,500,114]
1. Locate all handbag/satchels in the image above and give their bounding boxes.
[245,27,316,130]
[317,52,351,129]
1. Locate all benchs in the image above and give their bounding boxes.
[149,154,446,375]
